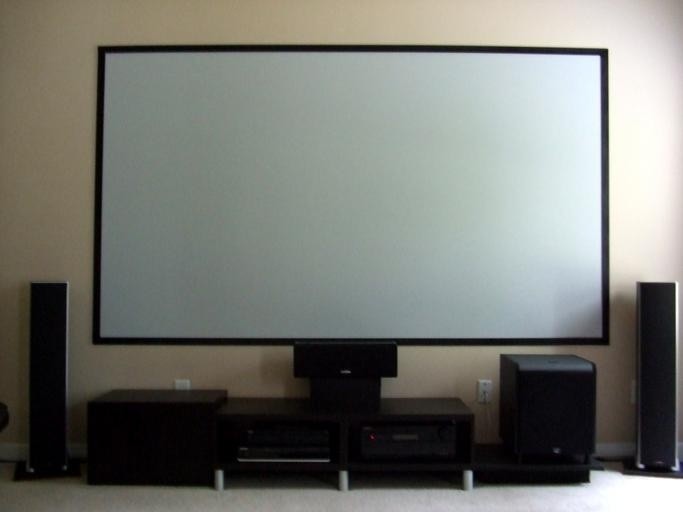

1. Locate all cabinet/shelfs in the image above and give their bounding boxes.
[84,390,476,491]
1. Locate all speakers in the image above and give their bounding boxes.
[498,353,596,465]
[12,283,83,481]
[621,281,682,476]
[294,340,397,411]
